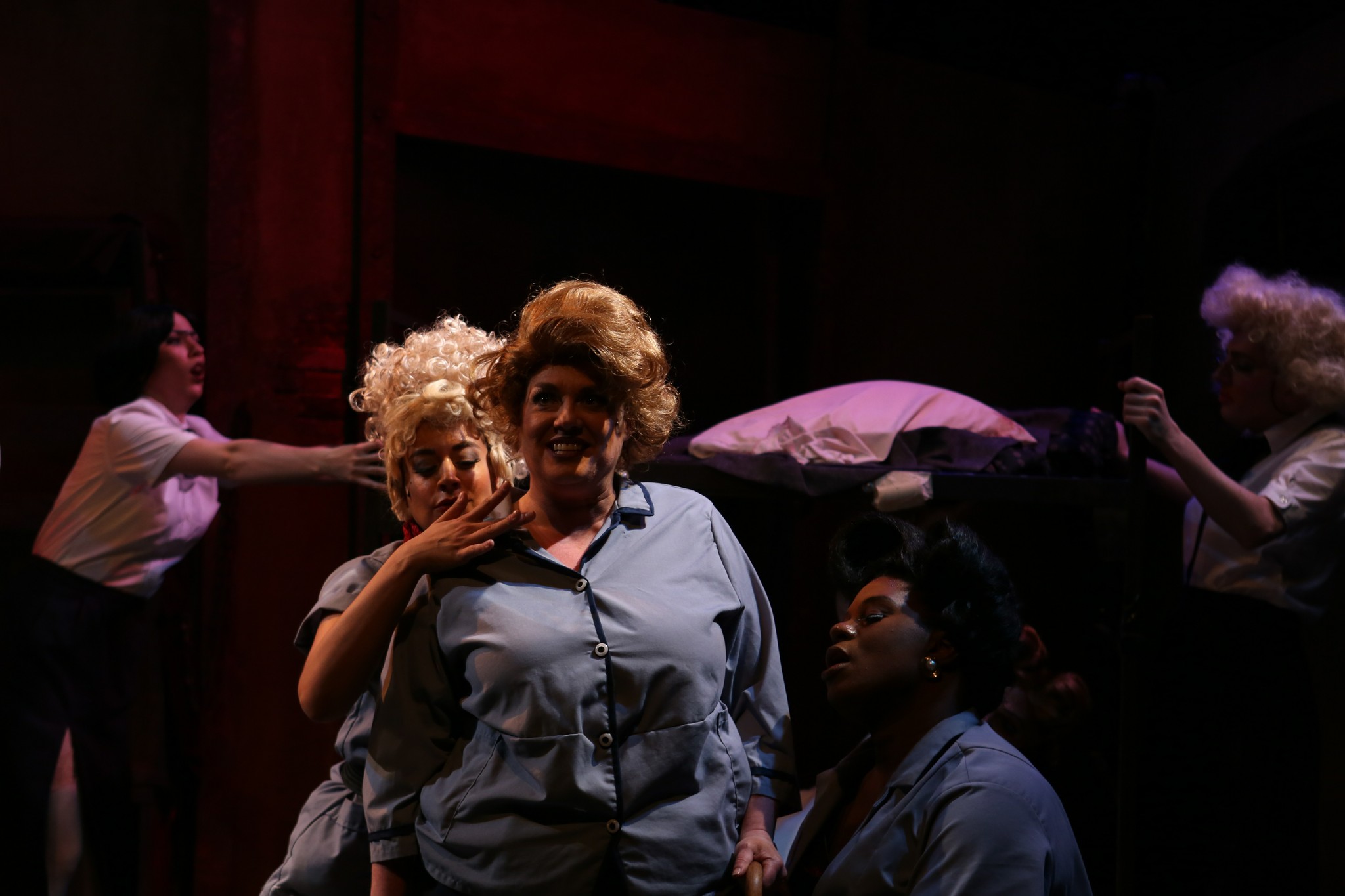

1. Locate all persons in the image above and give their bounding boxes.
[0,308,389,896]
[260,282,792,896]
[1092,263,1345,896]
[769,515,1093,896]
